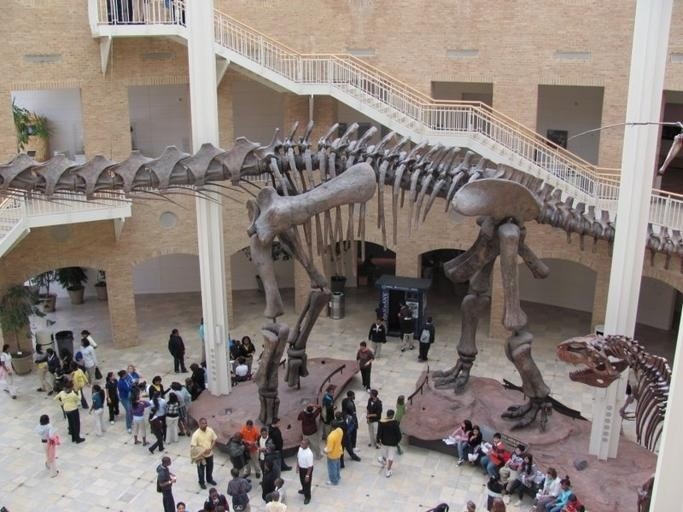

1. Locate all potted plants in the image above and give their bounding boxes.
[327,239,351,293]
[55,266,88,305]
[0,284,46,376]
[27,271,56,313]
[238,240,294,295]
[93,268,107,300]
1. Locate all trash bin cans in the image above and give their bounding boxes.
[328,290,346,321]
[55,331,74,360]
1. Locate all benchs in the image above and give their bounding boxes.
[513,460,585,512]
[473,424,529,494]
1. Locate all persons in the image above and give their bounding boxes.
[35,329,207,479]
[162,0,184,26]
[368,302,435,361]
[451,420,582,512]
[0,345,18,400]
[157,418,292,512]
[295,384,405,505]
[230,336,256,385]
[356,341,374,393]
